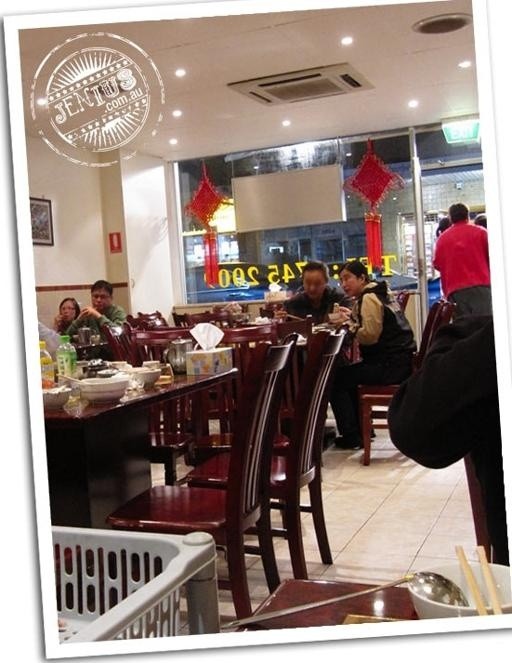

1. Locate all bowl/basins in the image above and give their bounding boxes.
[327,312,341,323]
[78,377,129,406]
[131,369,162,389]
[42,387,73,410]
[407,562,512,619]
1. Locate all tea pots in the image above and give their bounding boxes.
[163,336,199,374]
[78,325,97,346]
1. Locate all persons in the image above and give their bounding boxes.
[472,215,486,229]
[38,320,76,371]
[432,203,493,322]
[388,314,510,566]
[328,261,418,448]
[53,297,81,335]
[435,217,451,239]
[62,280,126,362]
[273,262,356,367]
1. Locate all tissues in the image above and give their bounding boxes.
[182,322,234,378]
[263,281,289,302]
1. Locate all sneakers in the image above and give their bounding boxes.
[333,440,363,449]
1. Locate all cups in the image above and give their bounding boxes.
[154,364,175,387]
[142,360,160,370]
[91,334,100,344]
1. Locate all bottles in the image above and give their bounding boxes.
[55,335,78,385]
[39,341,55,389]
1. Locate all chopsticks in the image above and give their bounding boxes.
[56,373,86,385]
[454,544,502,616]
[274,309,305,322]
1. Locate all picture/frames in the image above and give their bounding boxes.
[30,197,54,245]
